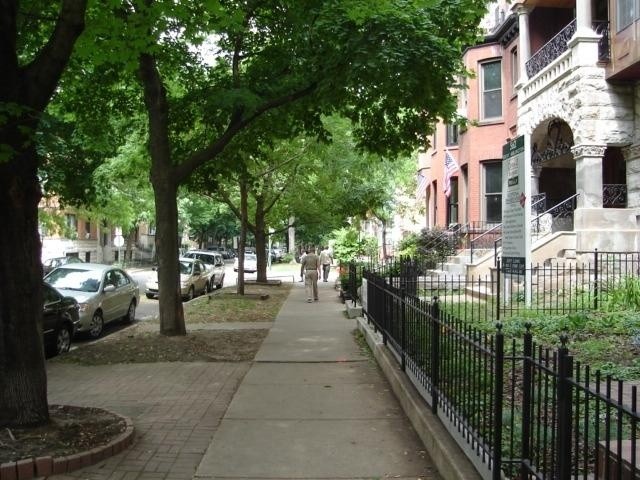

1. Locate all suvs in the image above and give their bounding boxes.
[183,249,225,292]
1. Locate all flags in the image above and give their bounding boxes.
[442,151,459,197]
[416,174,431,202]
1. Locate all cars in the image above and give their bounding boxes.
[146,259,209,301]
[209,242,285,273]
[42,256,140,357]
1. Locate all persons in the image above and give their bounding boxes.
[299,246,334,304]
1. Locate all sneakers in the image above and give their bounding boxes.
[308,299,313,303]
